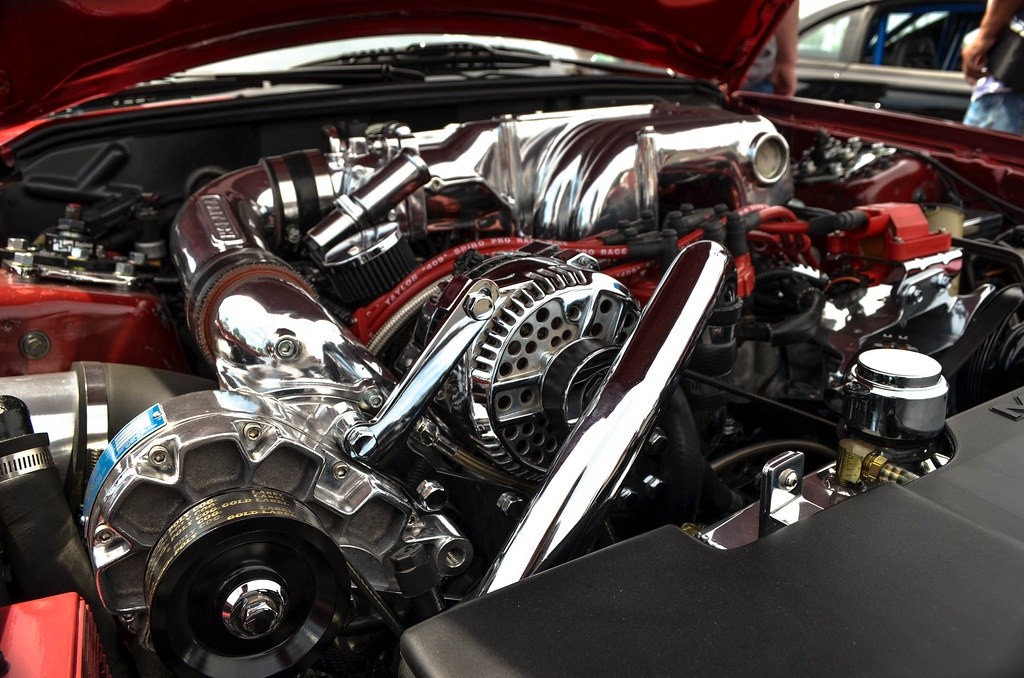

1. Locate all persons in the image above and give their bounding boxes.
[737,0,800,97]
[958,0,1024,86]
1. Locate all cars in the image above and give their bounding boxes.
[0,0,1023,677]
[741,0,1024,133]
